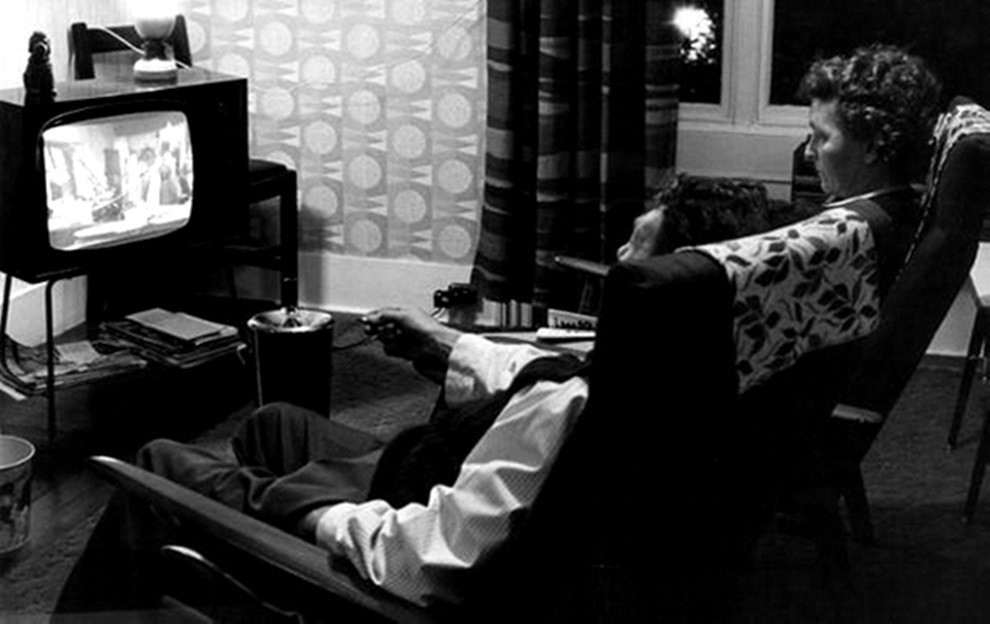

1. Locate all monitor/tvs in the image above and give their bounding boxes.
[0,64,256,283]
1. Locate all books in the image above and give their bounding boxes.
[108,307,240,369]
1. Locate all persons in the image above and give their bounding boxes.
[798,47,942,285]
[135,169,772,610]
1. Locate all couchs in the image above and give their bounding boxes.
[81,104,989,624]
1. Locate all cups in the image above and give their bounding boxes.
[0,433,36,552]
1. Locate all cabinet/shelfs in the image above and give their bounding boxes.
[0,158,306,466]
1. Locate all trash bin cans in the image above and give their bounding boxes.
[246,306,335,421]
[0,435,36,569]
